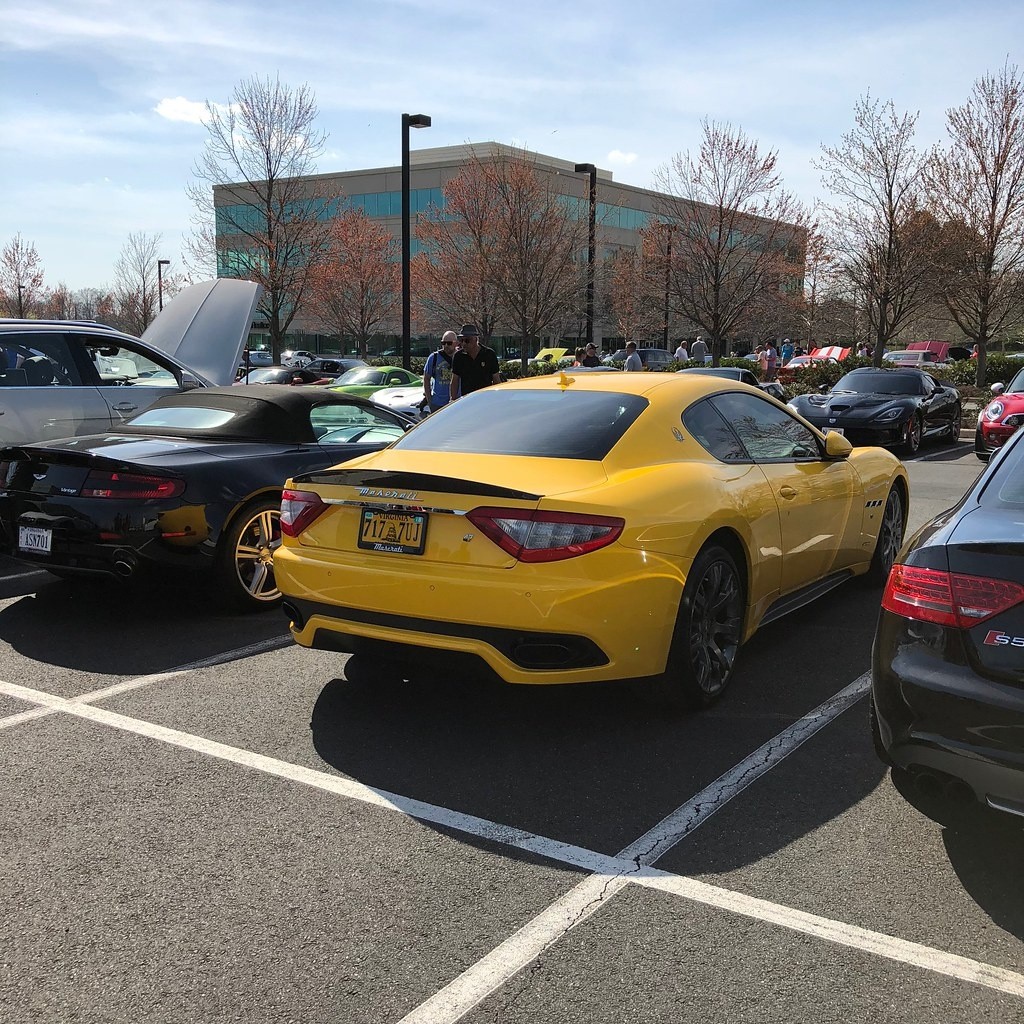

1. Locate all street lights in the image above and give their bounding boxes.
[398,108,435,373]
[156,258,172,314]
[573,162,597,346]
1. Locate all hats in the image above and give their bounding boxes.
[458,325,480,335]
[586,343,598,351]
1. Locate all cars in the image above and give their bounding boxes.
[498,343,983,365]
[237,345,430,446]
[1,383,428,623]
[277,370,909,710]
[976,364,1024,462]
[785,360,965,461]
[674,366,791,406]
[865,421,1024,826]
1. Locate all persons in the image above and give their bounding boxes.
[574,346,587,367]
[751,344,769,371]
[690,336,709,365]
[242,349,254,367]
[969,344,978,358]
[449,324,500,404]
[764,342,778,372]
[855,341,889,360]
[791,335,833,358]
[624,341,643,373]
[581,342,602,368]
[598,347,613,363]
[423,329,460,414]
[782,339,793,368]
[674,340,689,361]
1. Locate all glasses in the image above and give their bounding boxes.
[460,338,475,343]
[440,340,457,345]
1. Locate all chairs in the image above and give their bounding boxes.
[21,355,54,386]
[0,352,10,375]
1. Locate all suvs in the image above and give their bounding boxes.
[1,277,265,492]
